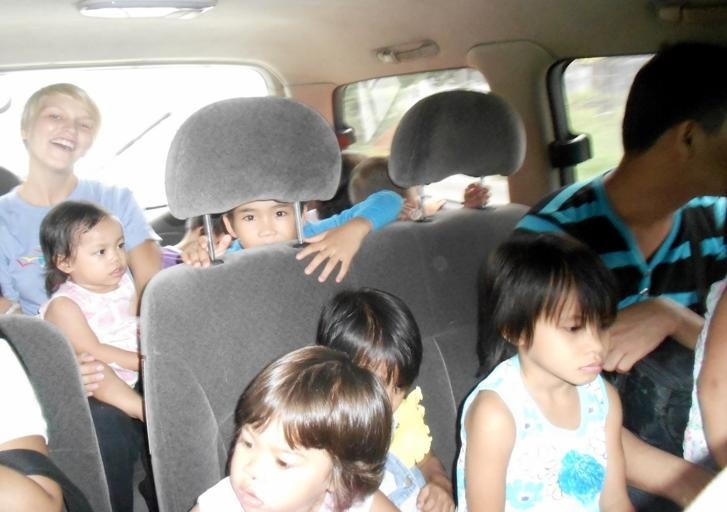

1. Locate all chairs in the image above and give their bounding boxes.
[2,311,116,512]
[2,166,24,196]
[137,95,460,511]
[392,90,532,416]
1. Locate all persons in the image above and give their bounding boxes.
[457,38,726,512]
[191,287,455,512]
[0,84,163,511]
[160,155,493,283]
